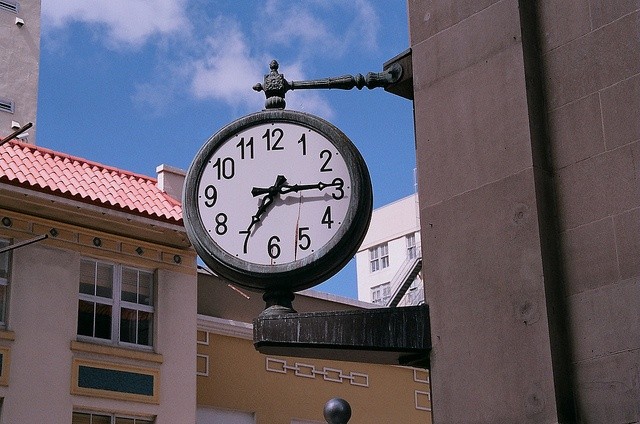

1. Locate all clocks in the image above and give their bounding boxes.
[181,108,373,293]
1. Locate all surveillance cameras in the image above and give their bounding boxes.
[16,132,29,140]
